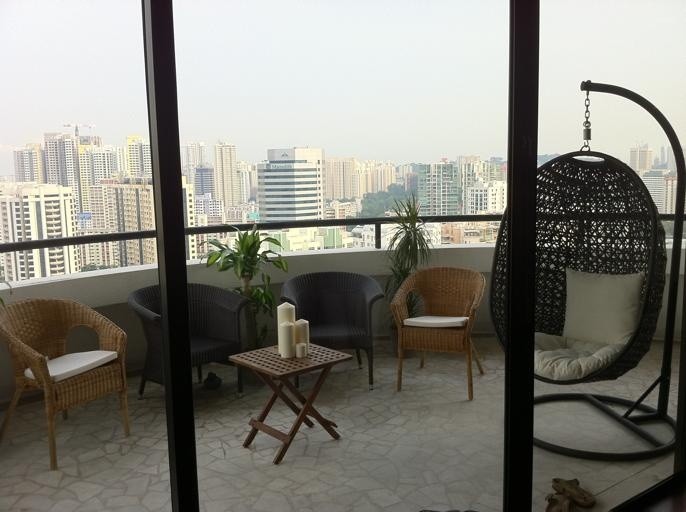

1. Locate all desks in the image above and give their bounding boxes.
[228,342,354,464]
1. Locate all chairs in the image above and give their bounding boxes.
[489,80,686,463]
[280,270,384,392]
[0,297,128,469]
[389,265,484,401]
[126,281,252,401]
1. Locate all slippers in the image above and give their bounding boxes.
[552,477,596,508]
[545,492,571,512]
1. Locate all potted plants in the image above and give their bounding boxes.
[196,223,288,387]
[381,192,432,359]
